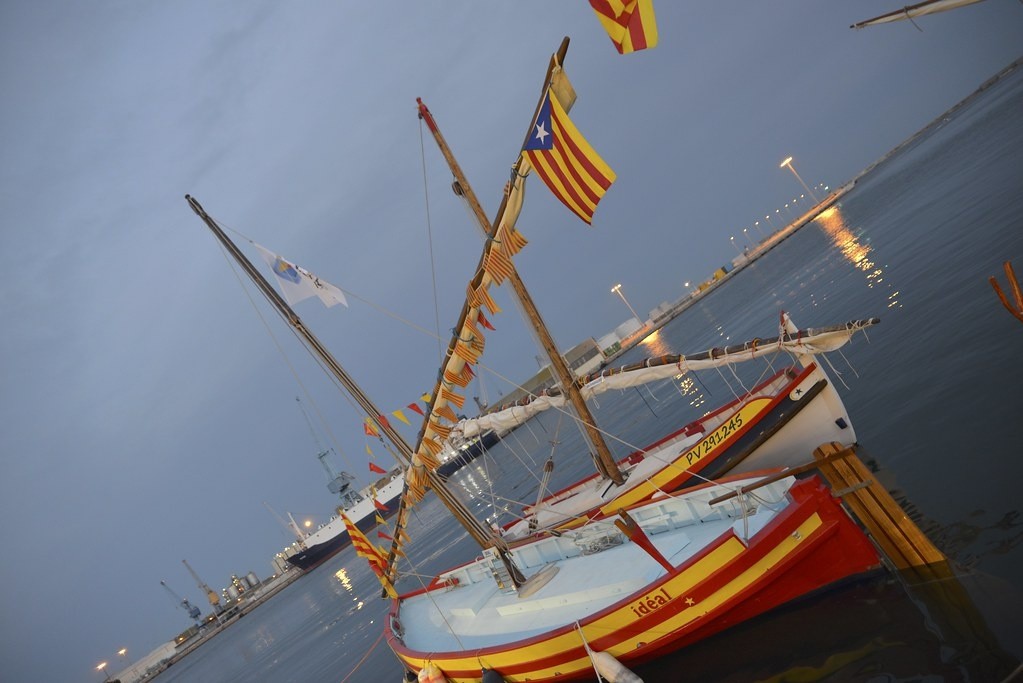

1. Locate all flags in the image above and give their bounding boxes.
[590,0,662,57]
[254,241,348,313]
[518,83,616,228]
[387,218,528,574]
[338,507,391,569]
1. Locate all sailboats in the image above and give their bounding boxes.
[184,35,947,683]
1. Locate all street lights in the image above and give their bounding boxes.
[610,284,645,328]
[779,157,819,204]
[119,648,138,677]
[96,662,110,679]
[785,204,795,222]
[730,236,741,254]
[766,215,778,233]
[754,222,767,239]
[775,209,787,226]
[743,228,756,249]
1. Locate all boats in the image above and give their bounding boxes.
[275,398,499,574]
